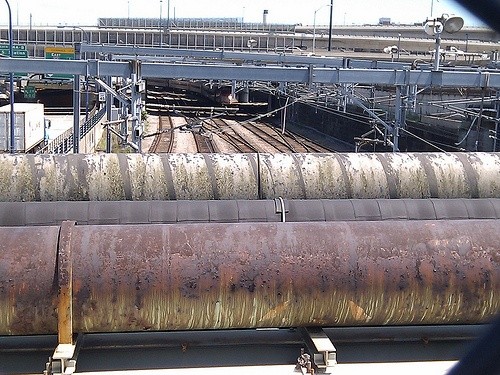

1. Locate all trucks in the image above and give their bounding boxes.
[0,103,51,155]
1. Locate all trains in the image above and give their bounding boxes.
[146,77,242,112]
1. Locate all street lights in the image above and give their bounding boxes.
[423,13,463,72]
